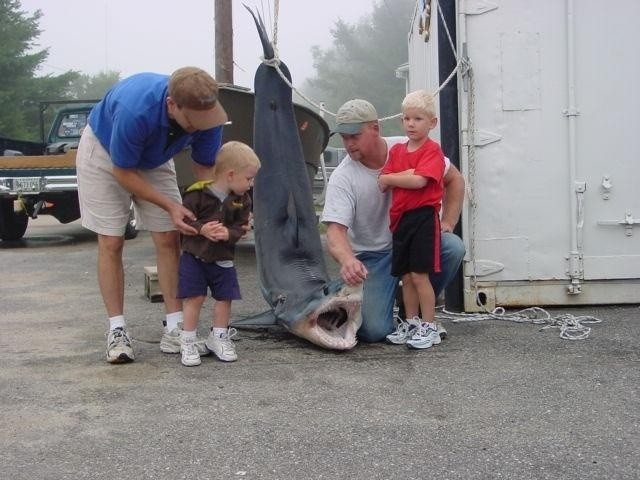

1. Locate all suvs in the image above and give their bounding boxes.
[0,100,139,242]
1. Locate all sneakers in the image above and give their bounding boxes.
[159,321,237,368]
[386,314,448,349]
[105,325,135,364]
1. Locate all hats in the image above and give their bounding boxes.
[330,98,378,135]
[168,66,229,131]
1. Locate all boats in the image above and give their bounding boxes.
[171,81,330,218]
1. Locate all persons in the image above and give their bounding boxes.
[376,90,448,350]
[76,66,252,364]
[318,98,467,344]
[178,141,262,368]
